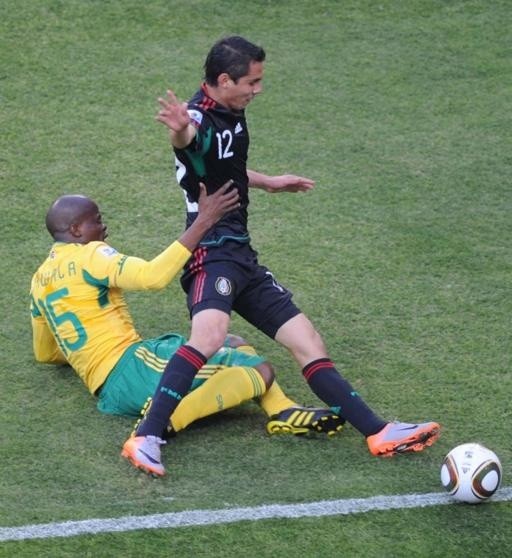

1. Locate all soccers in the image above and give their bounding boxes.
[441,443,502,505]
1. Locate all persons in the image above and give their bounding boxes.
[27,175,348,439]
[120,34,443,478]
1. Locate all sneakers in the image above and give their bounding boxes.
[264,402,347,438]
[366,420,441,457]
[119,432,168,479]
